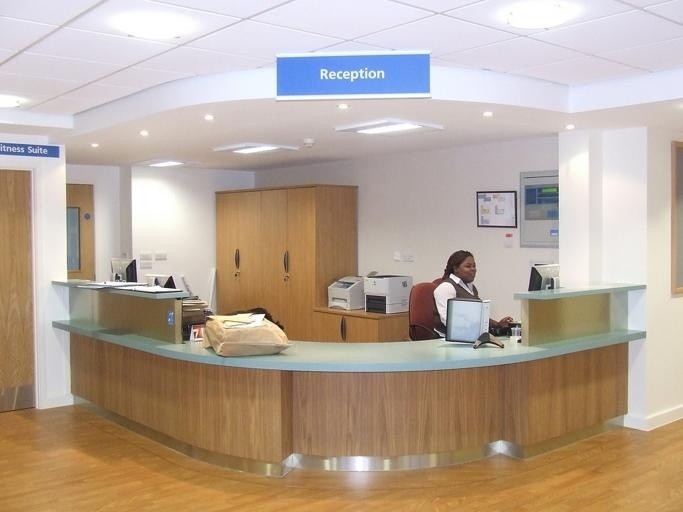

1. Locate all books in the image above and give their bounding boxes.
[182,296,216,342]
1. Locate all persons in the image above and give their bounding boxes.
[432,249,514,341]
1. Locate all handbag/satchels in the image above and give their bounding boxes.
[202,314,291,357]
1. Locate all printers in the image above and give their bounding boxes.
[328,275,365,311]
[365,275,413,315]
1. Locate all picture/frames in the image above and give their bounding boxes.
[477,190,518,229]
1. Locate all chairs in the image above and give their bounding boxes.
[408,282,444,339]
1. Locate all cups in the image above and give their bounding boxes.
[510,328,521,336]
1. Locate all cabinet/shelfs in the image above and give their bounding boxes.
[311,306,410,343]
[260,183,360,344]
[214,187,260,315]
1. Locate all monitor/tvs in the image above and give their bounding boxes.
[528,264,560,291]
